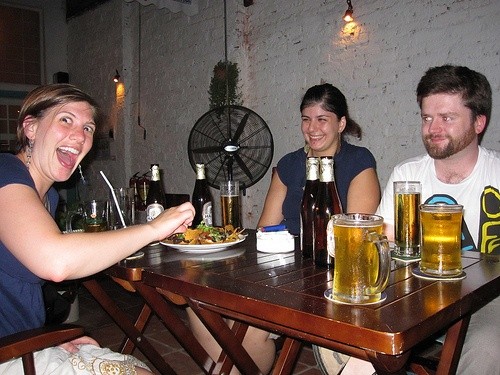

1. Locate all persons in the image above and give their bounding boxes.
[184,63,500,375]
[0,83,195,375]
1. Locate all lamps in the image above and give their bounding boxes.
[343,0,354,22]
[113,68,120,83]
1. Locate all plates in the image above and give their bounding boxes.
[160,233,246,254]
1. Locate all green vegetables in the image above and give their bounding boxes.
[177,224,223,242]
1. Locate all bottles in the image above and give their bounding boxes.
[299,157,321,269]
[146,164,166,224]
[312,156,345,271]
[190,162,213,230]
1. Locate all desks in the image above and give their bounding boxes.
[80,227,500,375]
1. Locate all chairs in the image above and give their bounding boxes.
[0,323,85,375]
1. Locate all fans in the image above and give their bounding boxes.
[188,106,274,191]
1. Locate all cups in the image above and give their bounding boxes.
[109,188,134,230]
[393,181,422,256]
[331,213,391,304]
[417,204,464,277]
[219,180,240,231]
[85,201,105,226]
